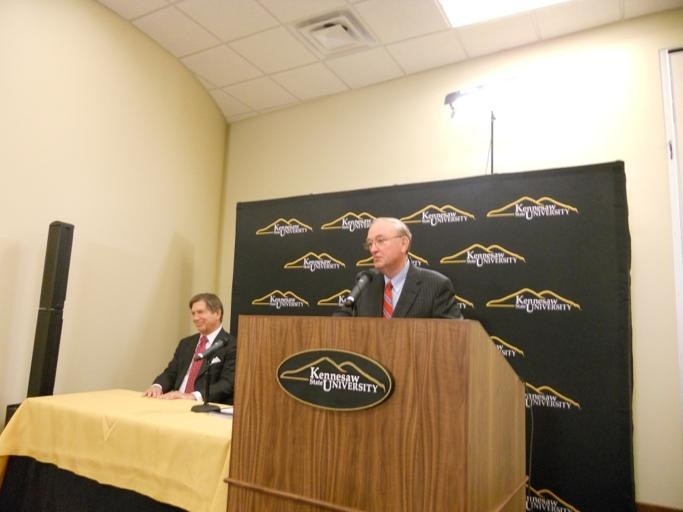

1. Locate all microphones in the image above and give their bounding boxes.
[345,271,373,308]
[194,336,230,361]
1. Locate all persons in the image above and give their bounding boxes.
[330,217,466,319]
[140,293,237,407]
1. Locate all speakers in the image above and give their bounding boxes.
[40,222,74,310]
[26,308,64,397]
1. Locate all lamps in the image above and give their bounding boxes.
[443,85,495,123]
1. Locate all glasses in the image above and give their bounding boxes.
[362,235,402,250]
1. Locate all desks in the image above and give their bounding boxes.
[0,389,233,512]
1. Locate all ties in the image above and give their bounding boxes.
[184,335,208,394]
[382,280,394,319]
